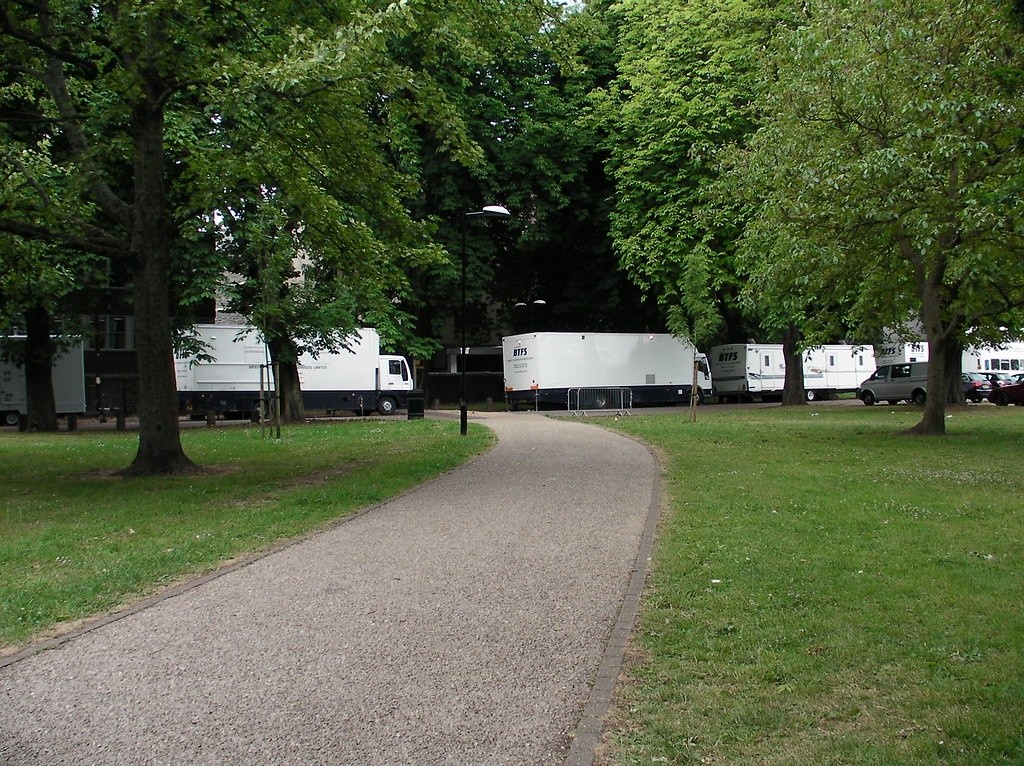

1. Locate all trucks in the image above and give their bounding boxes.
[873,341,982,375]
[502,332,713,410]
[172,324,414,422]
[0,334,87,427]
[709,344,888,404]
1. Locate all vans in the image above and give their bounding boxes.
[858,361,929,407]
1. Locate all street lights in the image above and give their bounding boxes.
[460,205,512,436]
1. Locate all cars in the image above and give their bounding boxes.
[986,374,1024,407]
[1011,374,1024,382]
[974,370,1019,390]
[962,372,993,404]
